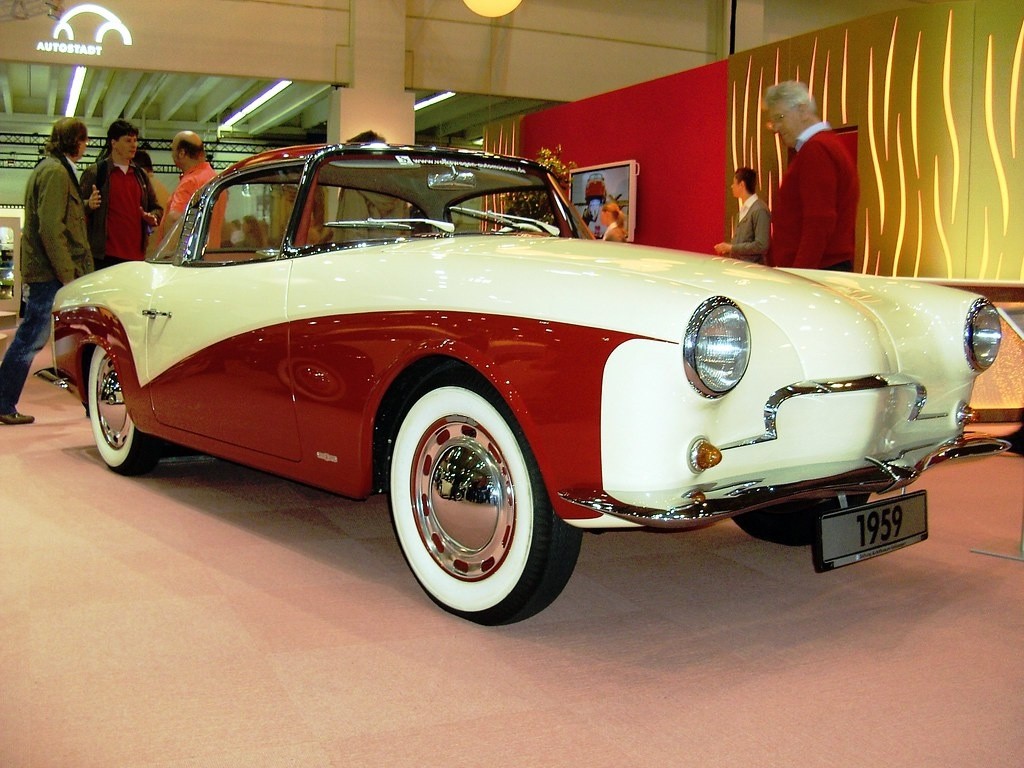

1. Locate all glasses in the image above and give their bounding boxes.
[767,103,798,129]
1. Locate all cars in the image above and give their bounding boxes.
[585,172,606,204]
[34,143,1013,626]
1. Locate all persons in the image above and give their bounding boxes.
[758,80,860,273]
[714,167,772,267]
[0,117,433,426]
[600,202,628,243]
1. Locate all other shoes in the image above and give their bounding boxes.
[0,412,35,425]
